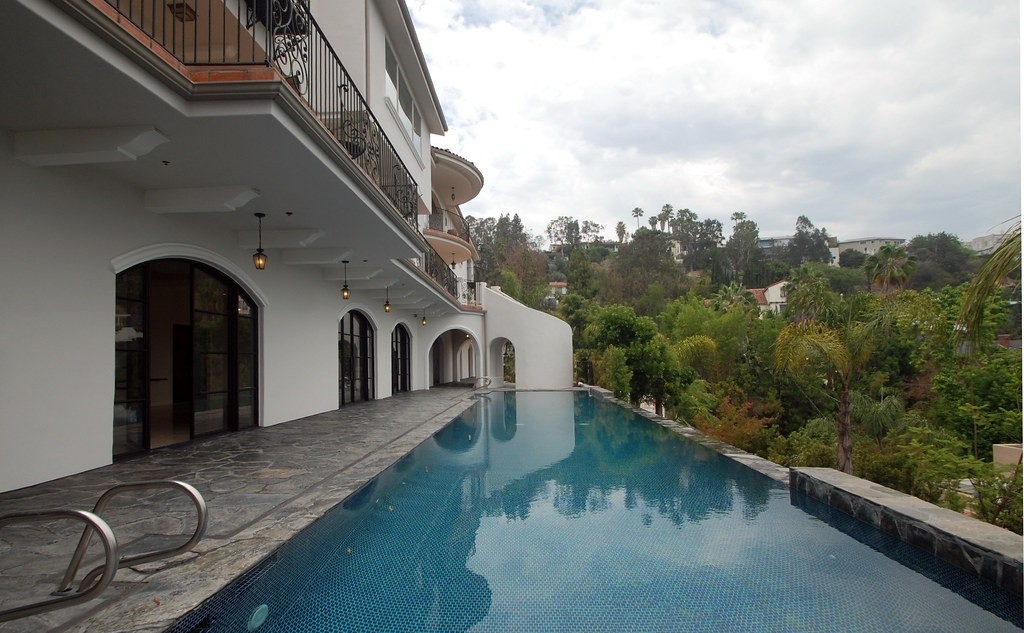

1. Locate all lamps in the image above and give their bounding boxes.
[253,212,268,270]
[422,309,426,325]
[451,187,455,201]
[167,2,197,22]
[341,260,351,299]
[451,252,456,269]
[384,285,391,313]
